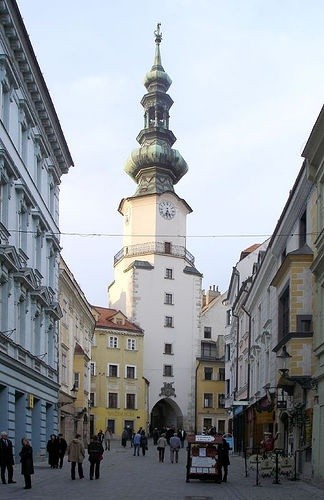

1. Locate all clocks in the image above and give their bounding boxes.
[158,200,176,220]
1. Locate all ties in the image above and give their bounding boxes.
[5,440,6,445]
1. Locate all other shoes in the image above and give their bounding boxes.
[12,481,16,483]
[23,487,31,489]
[216,481,221,484]
[80,476,84,479]
[222,479,226,482]
[3,482,6,484]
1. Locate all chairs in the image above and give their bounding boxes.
[206,443,217,457]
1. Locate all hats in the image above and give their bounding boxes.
[50,434,56,438]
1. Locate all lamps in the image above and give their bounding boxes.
[275,343,293,358]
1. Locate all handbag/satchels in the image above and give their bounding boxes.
[157,447,160,450]
[145,447,148,450]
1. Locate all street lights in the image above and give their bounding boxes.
[276,345,293,403]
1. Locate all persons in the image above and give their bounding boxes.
[87,435,104,480]
[140,432,148,456]
[55,433,67,469]
[206,443,216,449]
[157,433,167,463]
[0,431,17,485]
[170,433,181,464]
[19,436,34,489]
[97,424,186,451]
[133,431,142,458]
[67,434,86,480]
[46,434,56,469]
[218,434,230,482]
